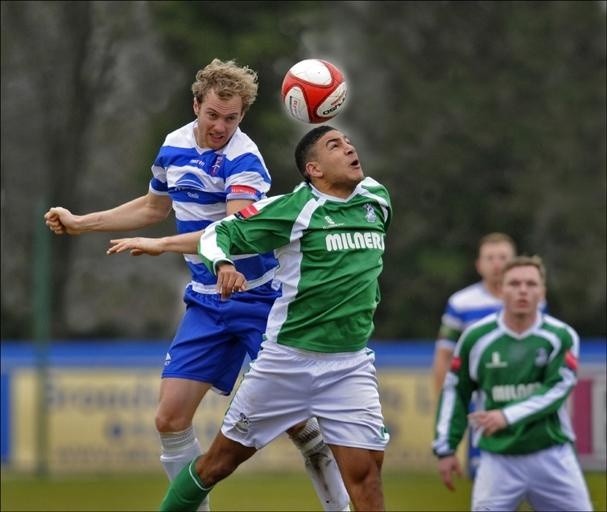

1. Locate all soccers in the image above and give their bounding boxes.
[282,59,347,123]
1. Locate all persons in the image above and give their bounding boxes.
[43,58,353,512]
[158,126,393,512]
[434,232,548,482]
[432,256,594,512]
